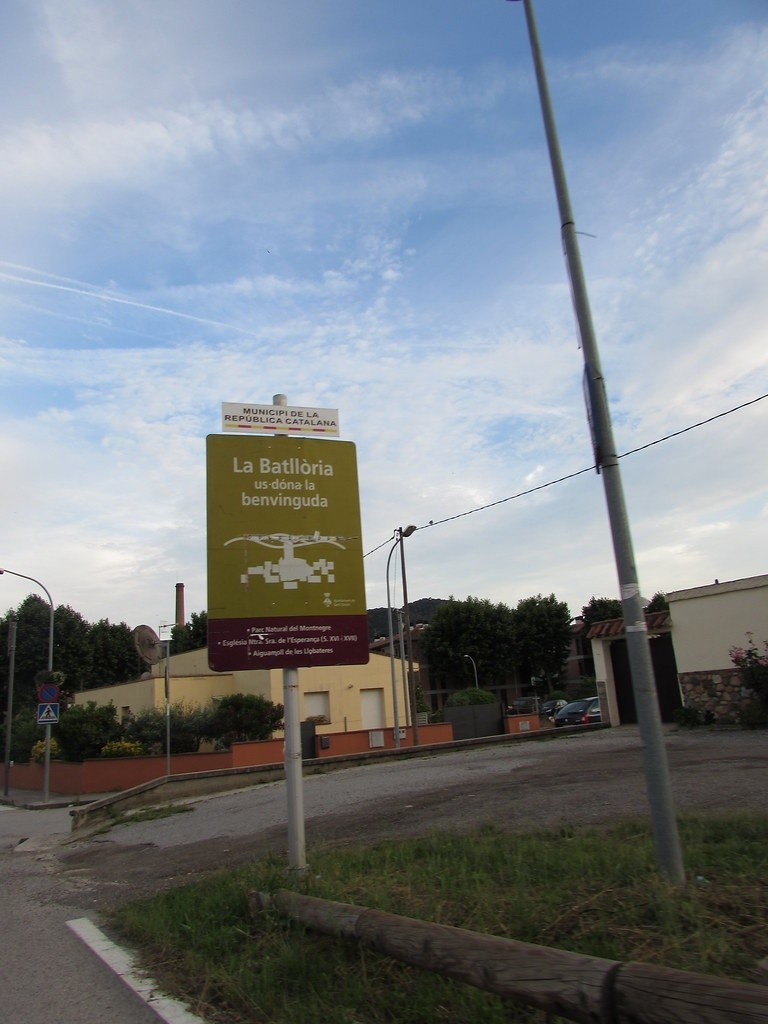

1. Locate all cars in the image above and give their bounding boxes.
[541,699,568,718]
[554,697,602,727]
[507,697,536,715]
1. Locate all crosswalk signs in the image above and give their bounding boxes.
[37,703,59,723]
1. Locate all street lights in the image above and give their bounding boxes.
[0,567,55,802]
[385,524,417,751]
[463,655,479,690]
